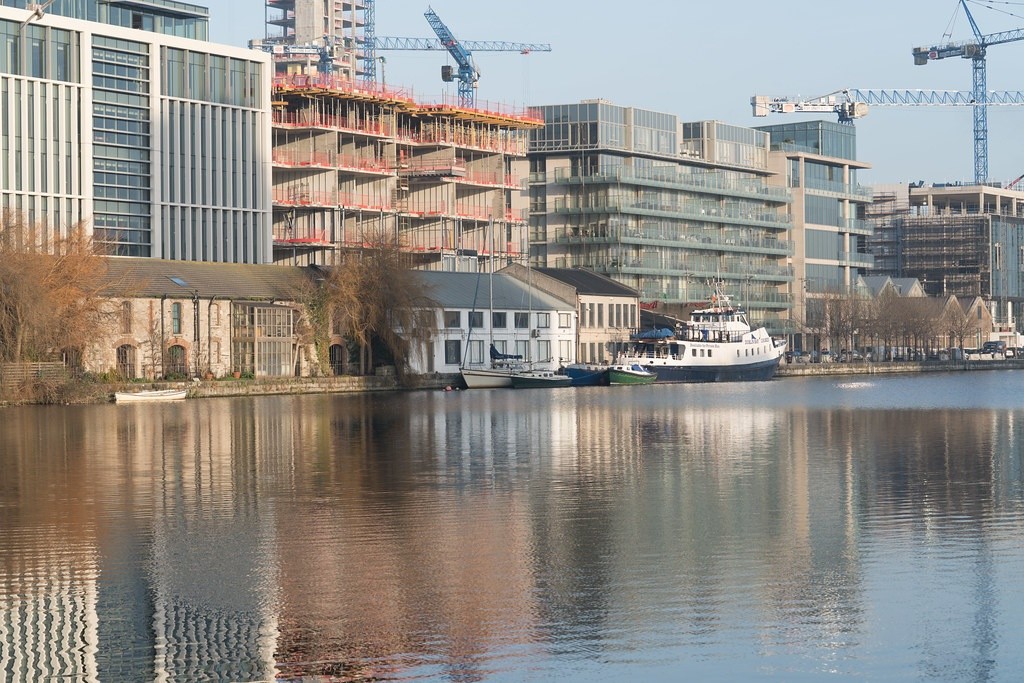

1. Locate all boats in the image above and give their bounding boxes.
[609,359,657,387]
[563,260,787,388]
[459,214,530,387]
[510,369,572,389]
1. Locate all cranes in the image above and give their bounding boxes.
[750,0,1023,187]
[322,6,552,109]
